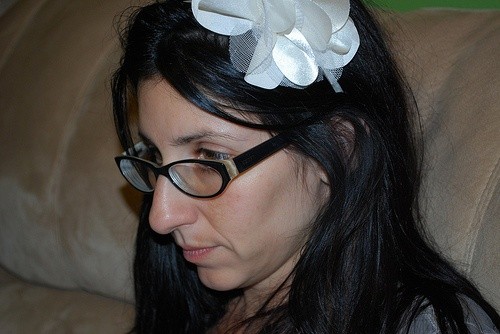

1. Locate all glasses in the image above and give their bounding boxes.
[115,133,294,198]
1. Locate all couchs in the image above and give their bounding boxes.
[0,0,500,334]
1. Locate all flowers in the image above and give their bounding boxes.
[191,0,361,93]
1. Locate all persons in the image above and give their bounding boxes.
[104,0,500,334]
